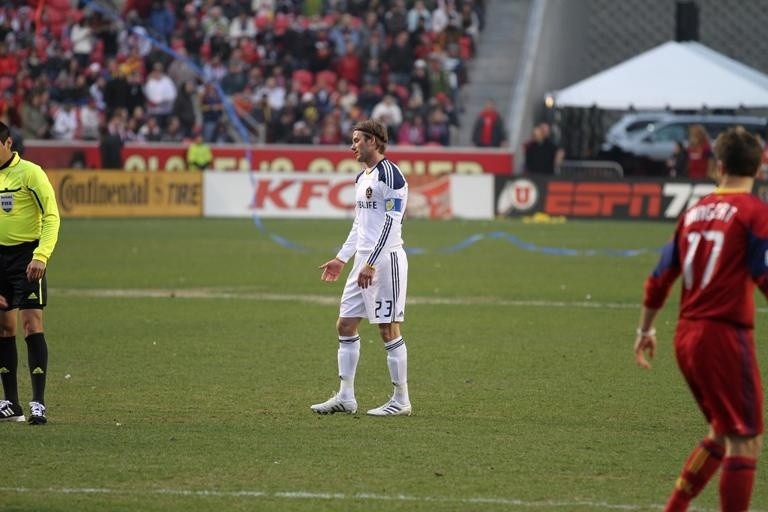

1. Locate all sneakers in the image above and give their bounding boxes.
[309,392,358,415]
[366,398,412,417]
[27,401,48,424]
[0,400,26,422]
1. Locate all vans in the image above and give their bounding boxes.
[605,111,767,176]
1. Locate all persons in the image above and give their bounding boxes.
[473,98,506,148]
[0,0,486,149]
[0,123,60,424]
[101,122,122,168]
[308,117,416,416]
[520,107,767,181]
[186,134,212,171]
[634,125,766,510]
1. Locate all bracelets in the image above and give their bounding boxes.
[634,326,656,339]
